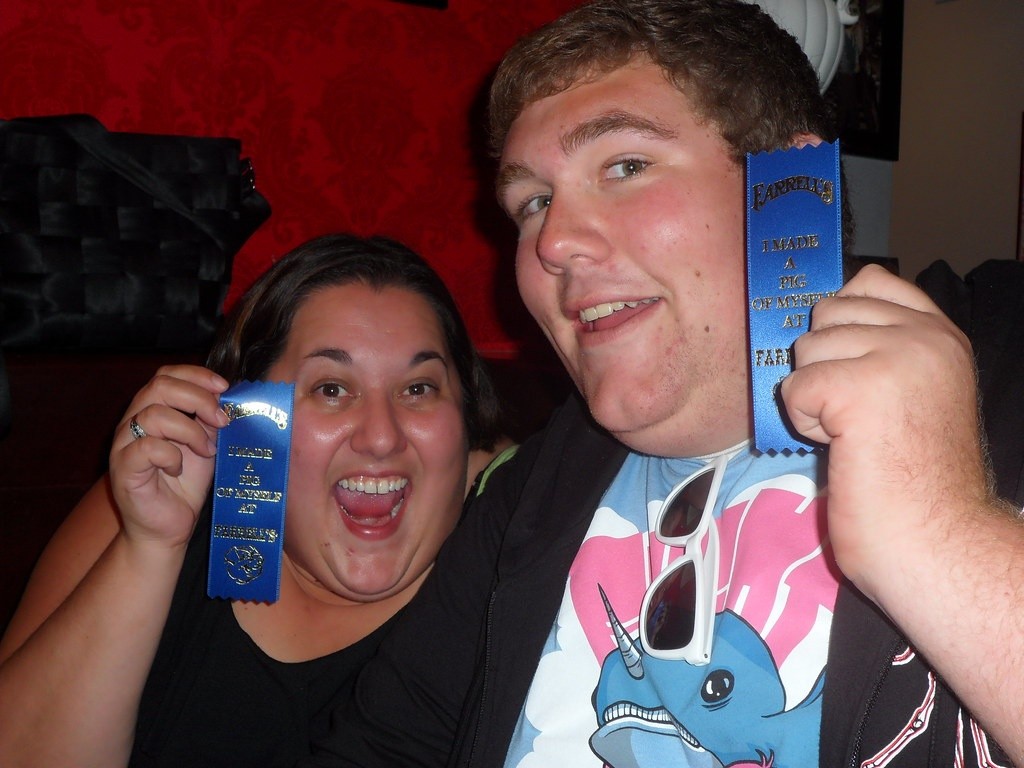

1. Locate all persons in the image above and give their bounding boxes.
[479,0,1023,768]
[0,233,517,768]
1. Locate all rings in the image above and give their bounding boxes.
[129,418,146,439]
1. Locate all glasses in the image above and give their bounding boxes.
[638,453,727,666]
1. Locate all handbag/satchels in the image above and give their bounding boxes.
[1,113,271,352]
